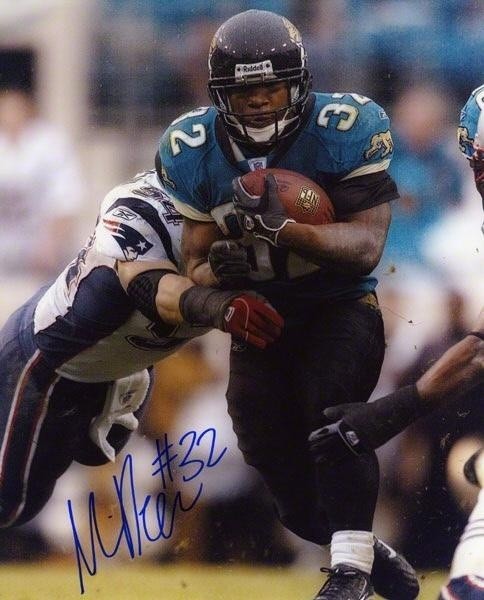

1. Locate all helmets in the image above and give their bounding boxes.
[208,10,313,149]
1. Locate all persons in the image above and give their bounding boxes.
[155,6,423,600]
[0,0,484,570]
[304,82,484,600]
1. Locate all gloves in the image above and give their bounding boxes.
[209,240,250,288]
[179,283,288,351]
[308,384,431,465]
[232,174,296,248]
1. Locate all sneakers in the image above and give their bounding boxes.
[314,537,419,600]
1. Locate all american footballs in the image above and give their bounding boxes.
[239,168,336,227]
